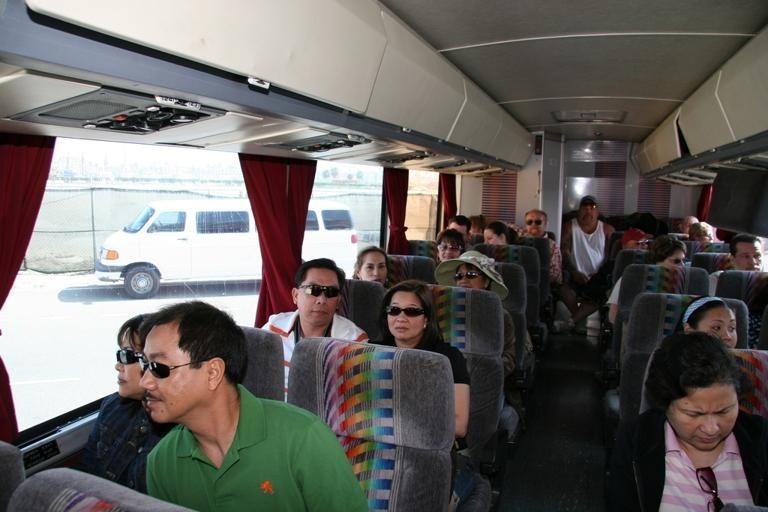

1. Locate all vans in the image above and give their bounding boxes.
[91,197,366,299]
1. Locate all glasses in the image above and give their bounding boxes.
[299,284,342,299]
[138,354,211,379]
[116,348,141,364]
[383,305,427,317]
[666,256,689,265]
[436,244,460,251]
[454,270,482,280]
[696,466,725,512]
[526,219,544,226]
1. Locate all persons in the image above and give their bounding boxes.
[87,195,767,510]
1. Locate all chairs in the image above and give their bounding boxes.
[1,209,765,509]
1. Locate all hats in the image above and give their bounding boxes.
[435,250,509,301]
[621,228,654,245]
